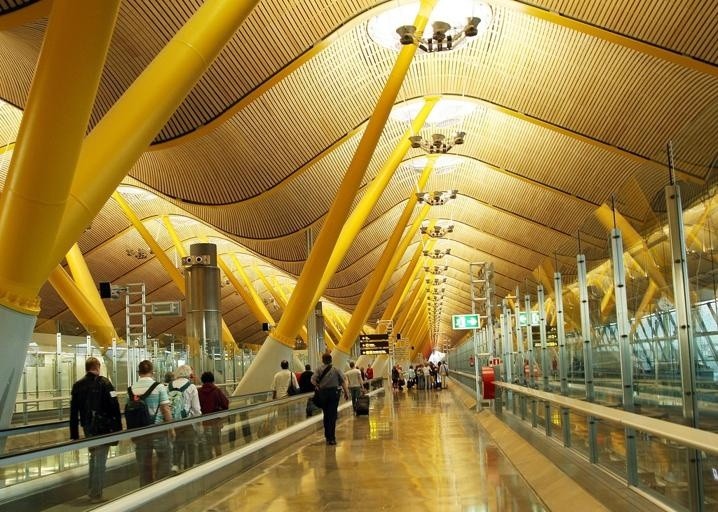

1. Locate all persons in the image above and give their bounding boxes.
[309,353,349,445]
[197,371,230,458]
[126,361,175,488]
[523,356,583,389]
[163,364,202,474]
[389,361,449,391]
[267,360,300,429]
[321,446,343,512]
[298,364,314,417]
[345,362,373,411]
[228,411,252,452]
[70,357,121,503]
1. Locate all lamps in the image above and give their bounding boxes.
[386,94,483,332]
[361,2,498,59]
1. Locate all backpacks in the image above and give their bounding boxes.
[125,382,160,443]
[167,381,191,423]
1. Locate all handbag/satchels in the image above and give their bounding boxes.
[313,384,321,408]
[287,385,301,396]
[88,410,118,446]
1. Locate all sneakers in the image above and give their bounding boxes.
[170,464,179,473]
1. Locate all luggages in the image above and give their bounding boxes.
[356,391,369,416]
